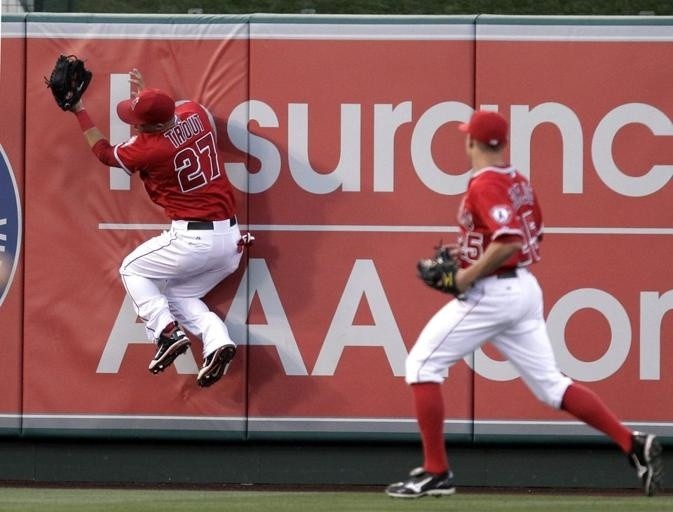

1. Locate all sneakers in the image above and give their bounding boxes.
[148,320,191,375]
[628,430,666,497]
[385,466,456,499]
[195,339,237,388]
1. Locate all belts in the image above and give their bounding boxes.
[187,219,236,231]
[498,268,517,279]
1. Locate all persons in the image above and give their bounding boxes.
[385,111,665,500]
[42,52,256,388]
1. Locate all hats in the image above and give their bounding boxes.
[457,110,508,148]
[117,87,175,125]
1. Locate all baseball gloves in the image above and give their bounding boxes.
[43,54,92,111]
[416,248,466,300]
